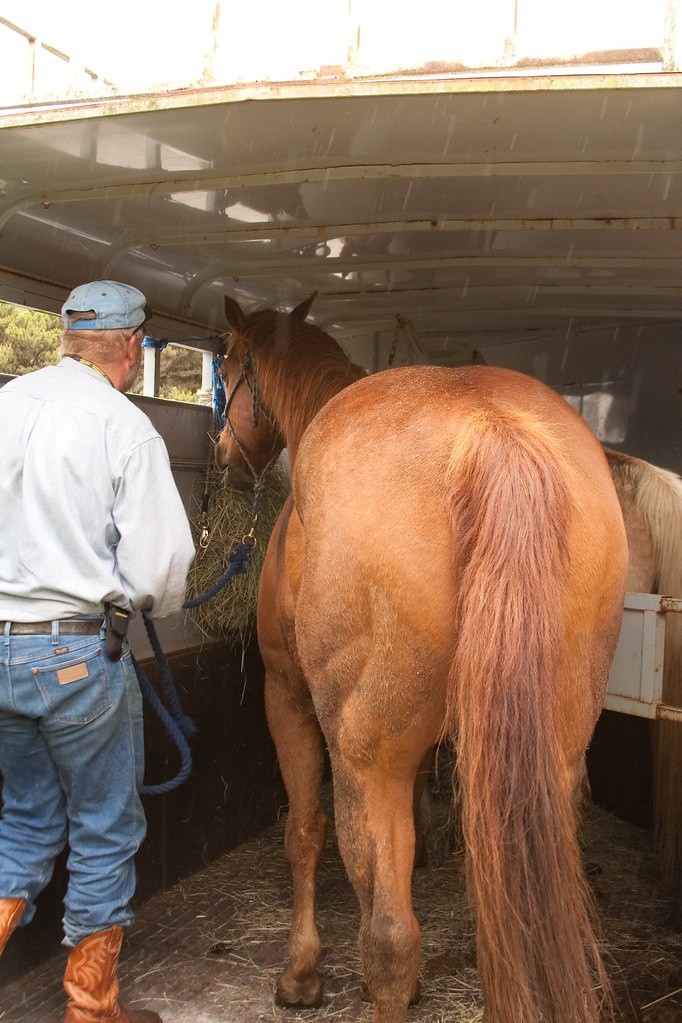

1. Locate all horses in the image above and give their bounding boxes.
[603,446,682,877]
[212,292,628,1023]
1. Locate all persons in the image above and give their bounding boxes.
[0,279,196,1023]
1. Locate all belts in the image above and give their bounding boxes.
[0,619,102,636]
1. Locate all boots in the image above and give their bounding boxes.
[0,897,28,953]
[63,924,162,1023]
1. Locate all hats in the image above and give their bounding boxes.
[61,279,151,330]
